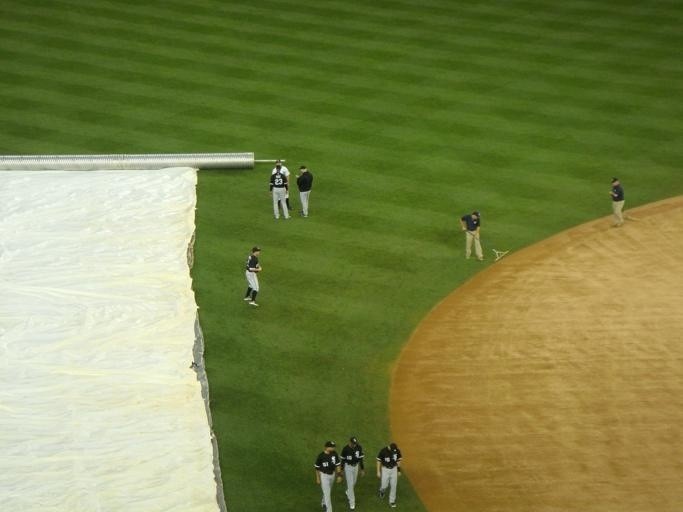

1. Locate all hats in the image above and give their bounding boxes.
[325,439,336,448]
[610,176,618,183]
[389,442,398,453]
[472,210,481,218]
[275,159,282,170]
[349,436,359,447]
[299,165,307,171]
[252,245,261,254]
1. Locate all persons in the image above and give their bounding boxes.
[294,164,314,218]
[608,176,625,227]
[458,210,485,261]
[339,437,365,511]
[374,444,403,509]
[242,245,263,308]
[313,440,342,512]
[269,164,292,219]
[271,160,293,210]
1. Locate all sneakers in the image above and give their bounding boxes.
[390,501,397,509]
[612,222,624,229]
[243,295,260,308]
[464,253,490,263]
[273,205,310,220]
[379,489,386,499]
